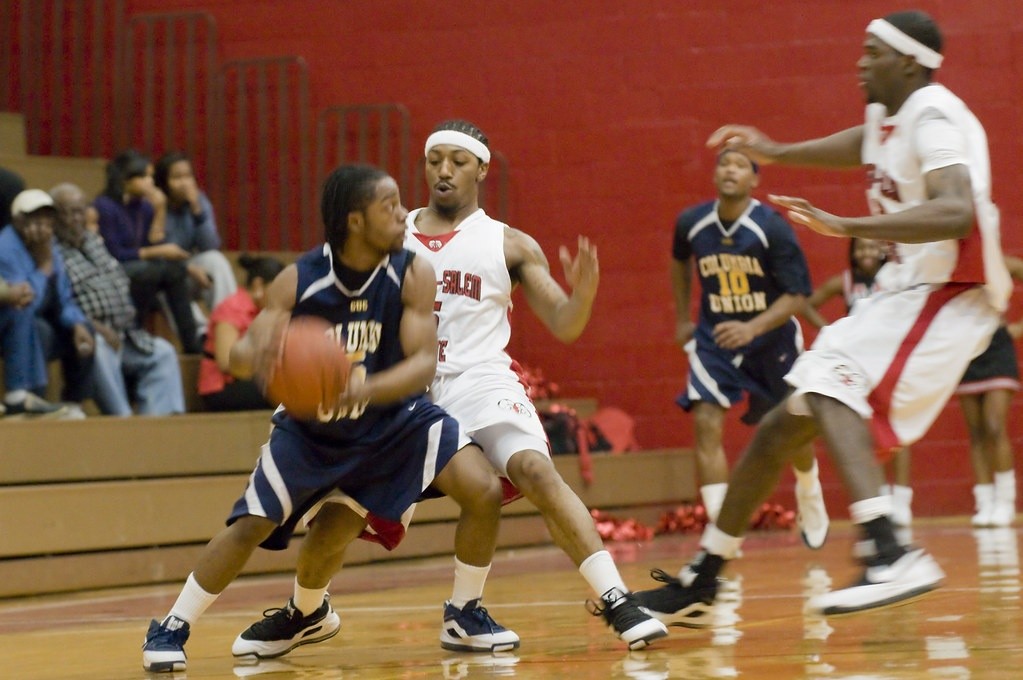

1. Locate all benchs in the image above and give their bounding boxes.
[0,113,708,598]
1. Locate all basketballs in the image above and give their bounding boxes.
[261,316,349,424]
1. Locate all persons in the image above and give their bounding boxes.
[668,146,830,560]
[0,169,187,421]
[91,151,206,353]
[803,232,914,526]
[950,253,1023,529]
[195,250,289,414]
[147,154,238,342]
[628,12,1017,626]
[140,165,522,673]
[231,117,668,662]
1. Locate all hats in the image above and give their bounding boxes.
[50,183,87,210]
[12,188,54,218]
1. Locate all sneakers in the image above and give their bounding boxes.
[233,663,343,680]
[631,567,725,630]
[232,591,340,660]
[794,479,830,549]
[583,586,668,652]
[623,653,669,676]
[141,615,191,672]
[821,546,944,616]
[725,549,742,561]
[439,597,520,652]
[440,654,520,678]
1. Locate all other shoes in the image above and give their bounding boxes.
[184,338,204,354]
[3,389,67,422]
[972,496,1015,528]
